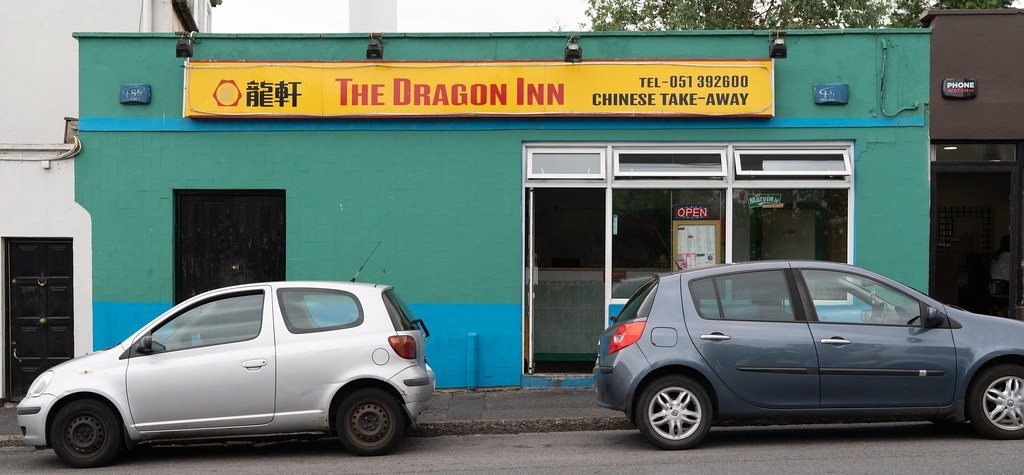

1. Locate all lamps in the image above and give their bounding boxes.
[769,30,787,59]
[366,32,384,58]
[564,34,582,63]
[175,30,197,58]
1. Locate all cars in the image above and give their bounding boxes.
[591,260,1024,449]
[14,280,435,469]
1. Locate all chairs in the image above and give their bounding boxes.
[750,276,790,321]
